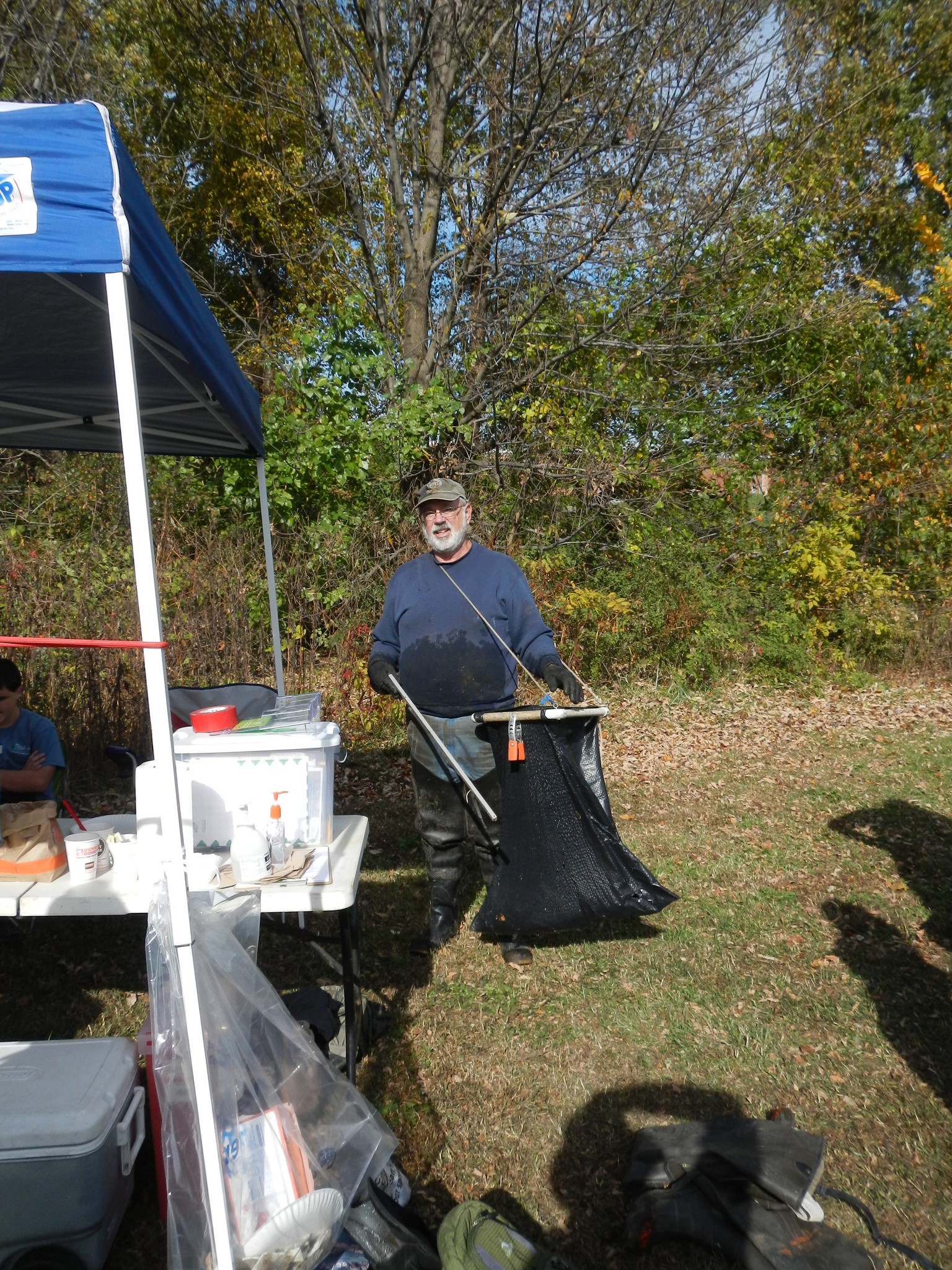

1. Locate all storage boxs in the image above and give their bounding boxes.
[173,721,340,849]
[0,1038,146,1270]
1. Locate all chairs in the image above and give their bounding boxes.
[108,683,278,789]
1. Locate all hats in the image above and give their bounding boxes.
[416,478,468,513]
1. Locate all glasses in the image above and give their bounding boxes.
[419,504,465,521]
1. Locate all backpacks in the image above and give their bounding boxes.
[436,1200,558,1270]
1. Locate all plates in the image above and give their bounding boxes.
[70,814,137,836]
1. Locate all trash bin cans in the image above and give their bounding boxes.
[472,704,679,940]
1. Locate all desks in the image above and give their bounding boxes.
[0,815,368,1102]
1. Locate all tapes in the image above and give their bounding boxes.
[190,705,239,732]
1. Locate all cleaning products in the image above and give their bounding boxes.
[230,802,274,881]
[266,789,289,865]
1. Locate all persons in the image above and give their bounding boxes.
[368,477,584,967]
[0,657,65,819]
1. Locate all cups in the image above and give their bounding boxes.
[63,833,101,882]
[106,833,138,883]
[80,822,114,871]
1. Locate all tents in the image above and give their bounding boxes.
[0,104,282,1270]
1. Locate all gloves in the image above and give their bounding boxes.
[367,661,402,701]
[542,664,584,705]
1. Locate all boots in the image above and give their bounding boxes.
[458,764,534,966]
[410,756,476,954]
